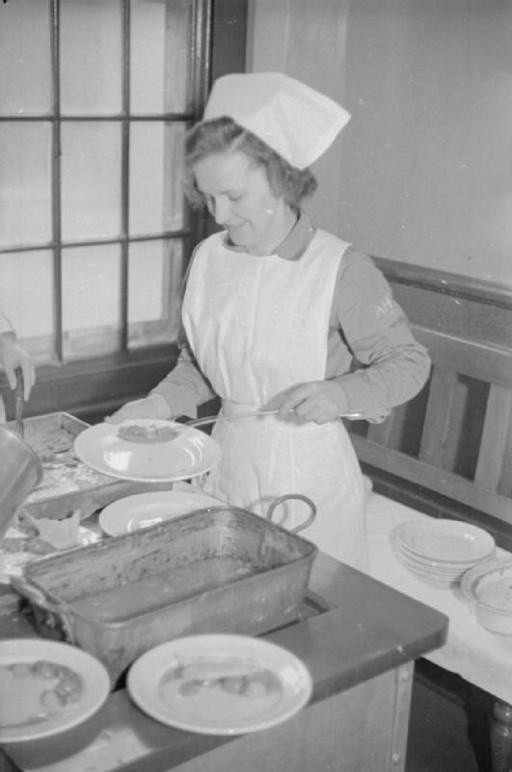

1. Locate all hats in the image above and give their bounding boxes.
[203,72,351,172]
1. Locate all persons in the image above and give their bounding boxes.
[103,115,431,576]
[0,310,37,405]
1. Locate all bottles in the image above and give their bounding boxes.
[489,702,512,772]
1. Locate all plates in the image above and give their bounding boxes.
[126,631,314,738]
[71,418,223,483]
[99,490,222,539]
[392,517,512,633]
[1,640,114,745]
[1,522,101,590]
